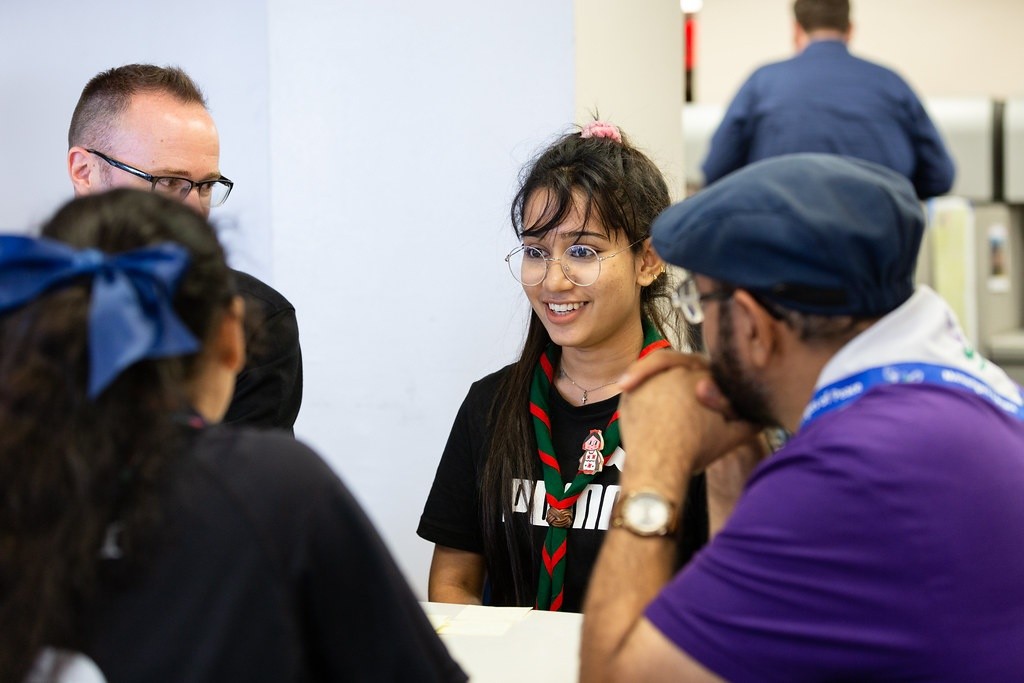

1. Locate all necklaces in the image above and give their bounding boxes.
[562,367,619,404]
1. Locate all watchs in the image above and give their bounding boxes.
[610,490,679,537]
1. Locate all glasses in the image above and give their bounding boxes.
[678,278,783,327]
[504,237,645,287]
[82,148,234,208]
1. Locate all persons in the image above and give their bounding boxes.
[576,0,1024,683]
[0,65,470,683]
[417,113,711,614]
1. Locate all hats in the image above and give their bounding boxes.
[650,151,926,316]
